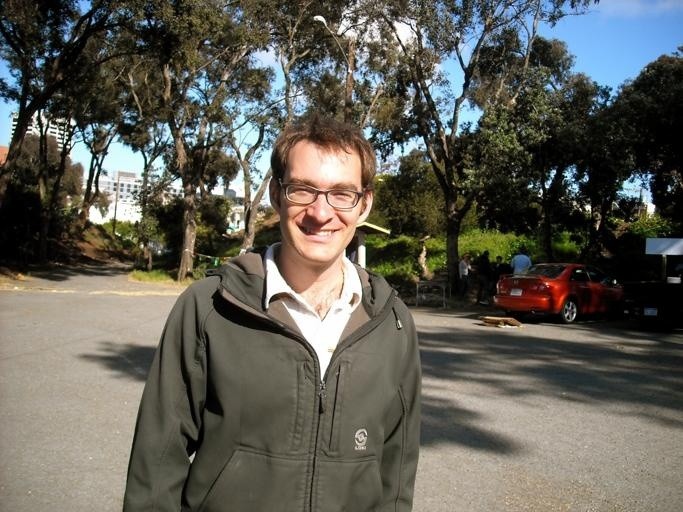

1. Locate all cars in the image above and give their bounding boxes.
[493,259,683,328]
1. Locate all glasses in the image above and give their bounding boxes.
[278,178,365,209]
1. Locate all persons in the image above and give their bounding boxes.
[459,247,532,302]
[121,112,421,512]
